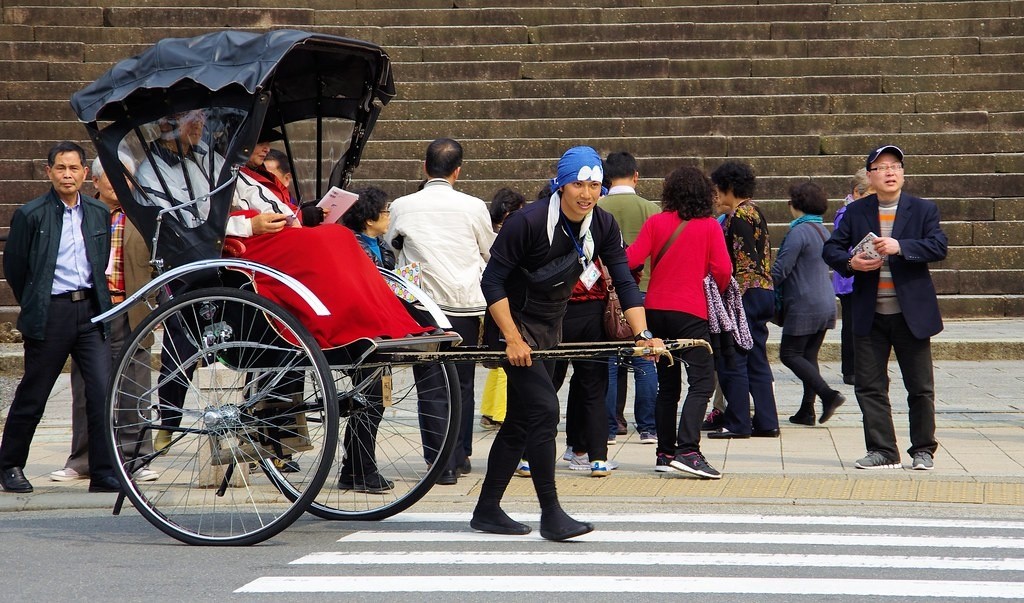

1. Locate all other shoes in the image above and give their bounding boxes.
[153,429,172,454]
[457,457,471,474]
[273,457,301,472]
[248,459,258,474]
[843,375,856,385]
[480,415,502,431]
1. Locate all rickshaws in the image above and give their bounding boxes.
[70,27,712,548]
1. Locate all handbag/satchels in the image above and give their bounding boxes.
[604,285,634,338]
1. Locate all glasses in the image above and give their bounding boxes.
[378,209,389,216]
[871,164,903,173]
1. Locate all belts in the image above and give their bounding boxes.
[111,295,125,304]
[54,288,96,302]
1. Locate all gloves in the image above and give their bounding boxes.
[302,206,324,227]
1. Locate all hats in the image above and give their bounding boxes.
[866,145,904,172]
[229,122,287,146]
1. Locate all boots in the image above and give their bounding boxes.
[525,439,594,540]
[470,435,532,535]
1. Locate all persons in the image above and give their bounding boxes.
[469,146,665,540]
[822,146,948,470]
[134,109,226,227]
[49,150,159,481]
[0,141,125,493]
[223,127,847,492]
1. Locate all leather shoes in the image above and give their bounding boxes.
[0,466,33,493]
[88,476,122,492]
[436,468,457,485]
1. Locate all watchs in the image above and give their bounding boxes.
[634,330,653,343]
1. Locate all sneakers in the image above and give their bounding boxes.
[49,467,89,481]
[589,460,611,477]
[513,459,531,476]
[353,472,395,491]
[133,467,158,481]
[562,445,573,461]
[568,453,593,470]
[337,473,355,489]
[606,385,933,479]
